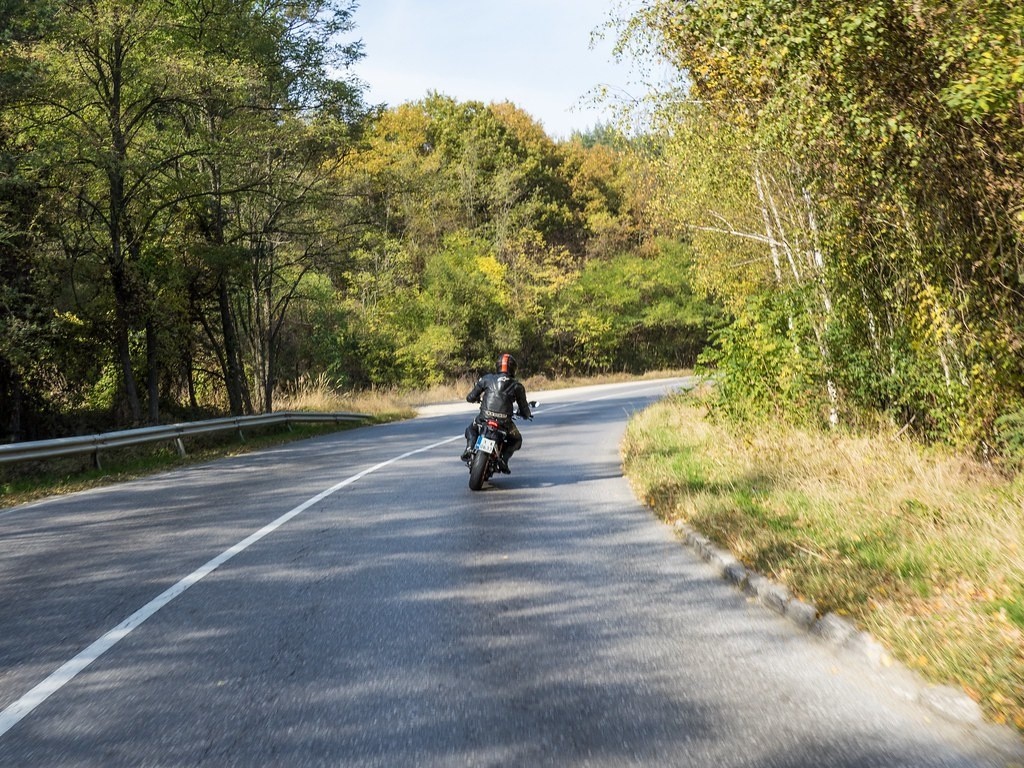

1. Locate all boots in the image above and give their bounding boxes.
[499,446,515,474]
[461,429,476,461]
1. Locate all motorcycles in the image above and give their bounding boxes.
[463,377,541,490]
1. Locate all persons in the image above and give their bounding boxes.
[461,354,530,475]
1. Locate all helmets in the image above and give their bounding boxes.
[496,354,518,377]
